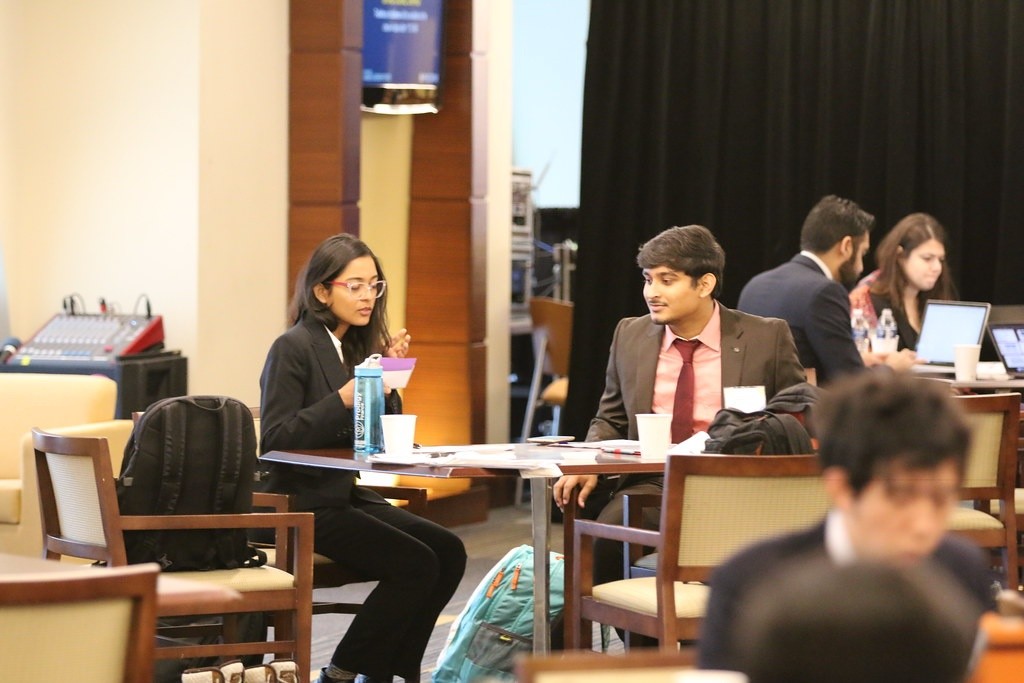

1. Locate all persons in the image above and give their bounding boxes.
[699,367,1024,683]
[258,232,467,683]
[550,225,807,655]
[848,214,959,365]
[738,194,930,388]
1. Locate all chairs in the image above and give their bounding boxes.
[32,407,428,683]
[515,367,1024,683]
[514,296,575,507]
[0,372,133,567]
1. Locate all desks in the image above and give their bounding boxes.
[949,378,1024,393]
[258,441,674,654]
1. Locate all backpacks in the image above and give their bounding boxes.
[701,407,815,459]
[93,393,268,572]
[432,542,565,683]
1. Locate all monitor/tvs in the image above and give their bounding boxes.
[362,0,446,115]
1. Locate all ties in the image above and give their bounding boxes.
[669,336,702,444]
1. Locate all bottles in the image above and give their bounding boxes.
[353,354,385,453]
[851,308,869,353]
[876,308,897,354]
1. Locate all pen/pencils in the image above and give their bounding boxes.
[604,449,635,454]
[428,453,455,458]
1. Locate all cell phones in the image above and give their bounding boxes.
[527,435,575,444]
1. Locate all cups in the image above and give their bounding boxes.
[951,345,982,381]
[635,413,673,459]
[381,414,417,456]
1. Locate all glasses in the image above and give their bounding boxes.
[321,277,386,300]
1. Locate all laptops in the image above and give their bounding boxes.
[911,298,991,378]
[986,323,1024,379]
